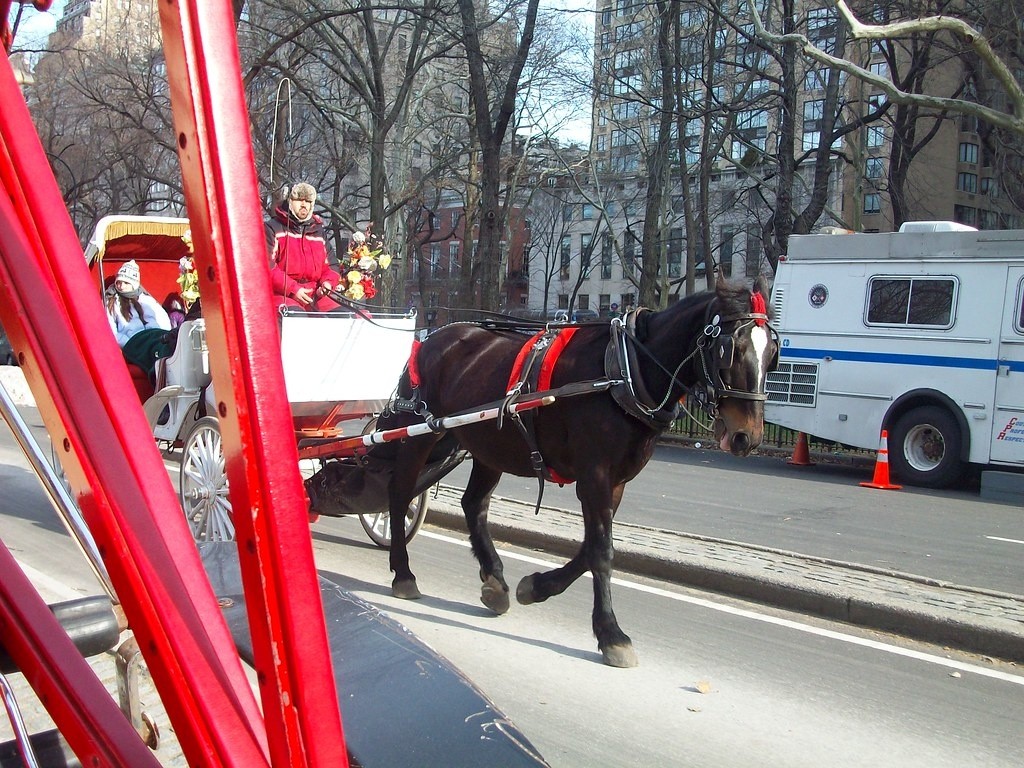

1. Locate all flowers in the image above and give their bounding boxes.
[176,231,199,311]
[335,223,392,300]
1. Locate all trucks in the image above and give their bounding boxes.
[762,220,1024,487]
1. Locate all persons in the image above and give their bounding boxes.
[264,181,356,318]
[104,259,202,426]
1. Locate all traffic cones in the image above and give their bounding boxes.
[787,430,817,466]
[860,430,904,491]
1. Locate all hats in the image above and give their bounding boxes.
[115,259,140,291]
[282,181,317,223]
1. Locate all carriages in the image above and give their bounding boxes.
[48,215,782,668]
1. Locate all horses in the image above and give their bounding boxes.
[374,266,779,668]
